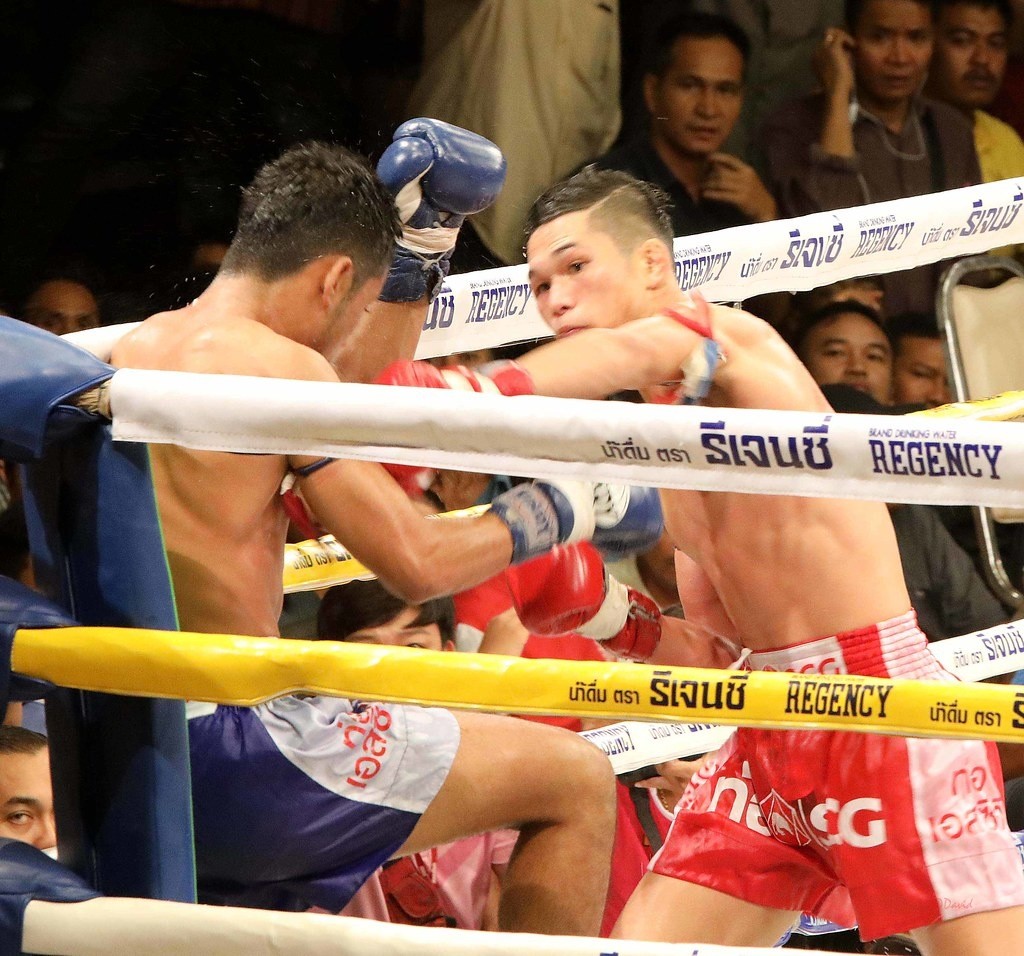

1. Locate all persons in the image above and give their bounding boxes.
[105,116,664,937]
[0,725,58,860]
[317,0,1024,941]
[372,167,1024,956]
[24,281,101,336]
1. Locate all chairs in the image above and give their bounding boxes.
[937,257,1024,608]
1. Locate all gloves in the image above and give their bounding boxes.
[369,361,537,397]
[488,468,665,568]
[375,117,506,301]
[505,542,661,662]
